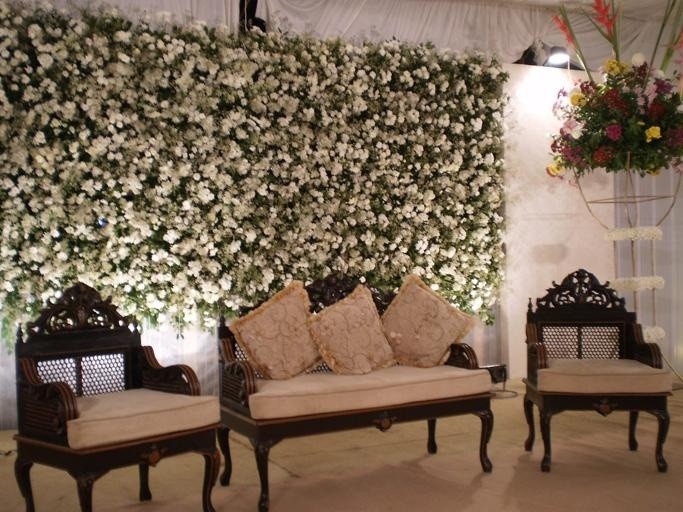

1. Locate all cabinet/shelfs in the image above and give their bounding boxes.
[571,149,683,392]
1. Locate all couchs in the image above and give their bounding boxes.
[217,272,496,511]
[13,282,223,512]
[523,269,673,473]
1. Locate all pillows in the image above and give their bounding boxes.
[379,273,475,367]
[228,280,322,380]
[307,283,396,376]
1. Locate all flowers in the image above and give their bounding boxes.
[545,0,683,187]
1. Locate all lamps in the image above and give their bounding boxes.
[548,46,569,66]
